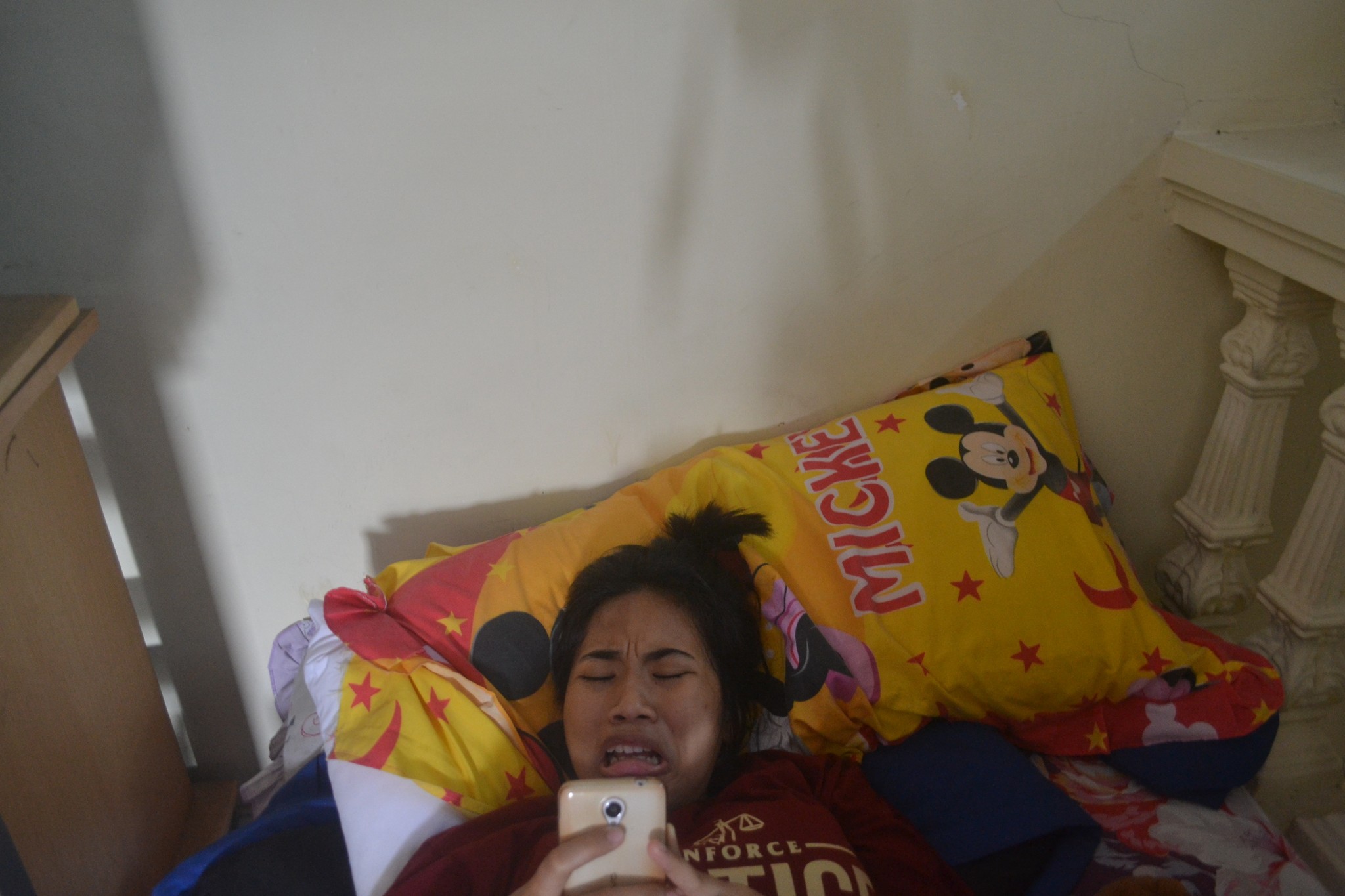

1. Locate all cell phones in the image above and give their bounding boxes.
[556,775,666,890]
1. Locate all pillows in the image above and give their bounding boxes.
[337,328,1225,829]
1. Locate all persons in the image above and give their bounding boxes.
[382,500,969,894]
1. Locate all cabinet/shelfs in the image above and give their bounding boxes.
[0,292,198,896]
